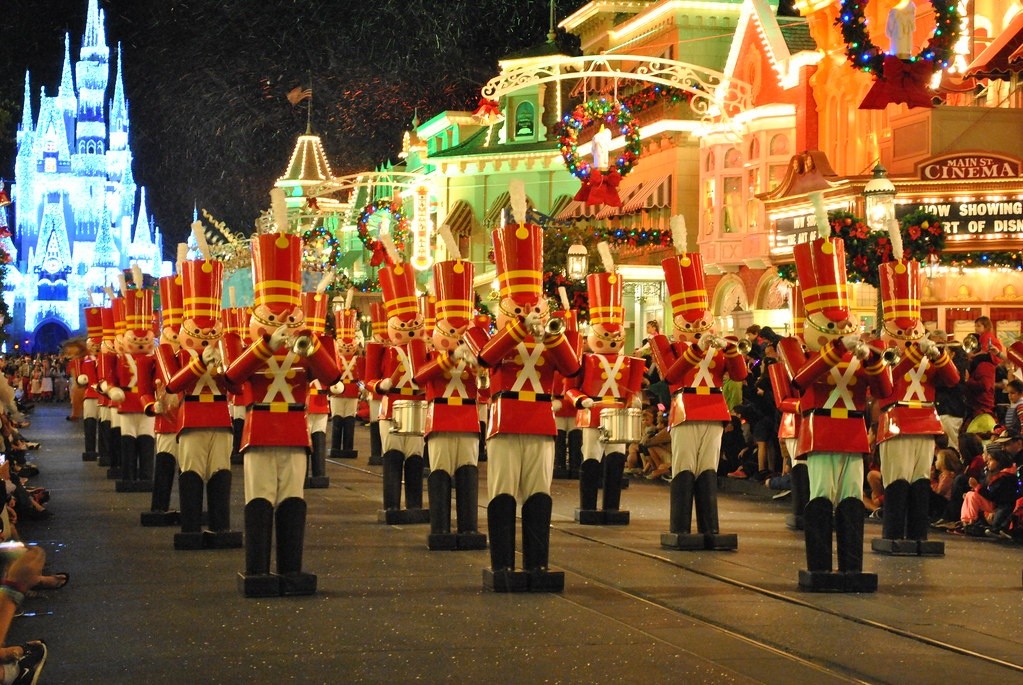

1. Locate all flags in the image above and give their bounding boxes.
[286,79,312,107]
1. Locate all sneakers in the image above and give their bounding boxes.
[727,471,748,478]
[12,643,47,685]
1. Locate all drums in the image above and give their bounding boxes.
[598,406,643,445]
[390,399,429,438]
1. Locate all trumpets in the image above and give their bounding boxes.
[282,333,315,358]
[850,340,903,367]
[935,333,980,354]
[708,334,753,356]
[211,349,231,375]
[529,315,567,339]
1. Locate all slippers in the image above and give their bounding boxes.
[30,573,69,590]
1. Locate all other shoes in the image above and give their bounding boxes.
[936,522,956,529]
[15,639,45,658]
[946,528,960,534]
[26,487,45,494]
[19,441,40,451]
[661,473,672,482]
[999,530,1018,540]
[772,489,792,501]
[870,509,883,520]
[33,491,50,503]
[27,404,34,410]
[18,422,29,427]
[930,519,945,529]
[32,502,45,513]
[984,529,1001,538]
[953,527,966,536]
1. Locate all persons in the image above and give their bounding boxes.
[627,316,1023,535]
[70,302,957,598]
[0,352,73,685]
